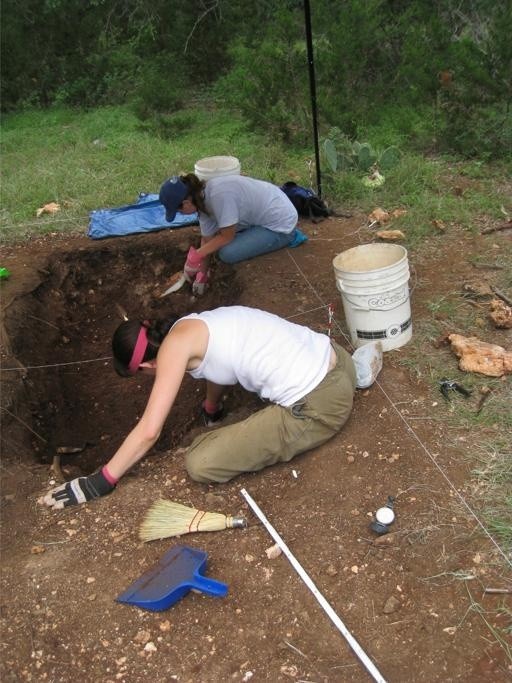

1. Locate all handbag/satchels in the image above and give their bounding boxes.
[279,180,330,224]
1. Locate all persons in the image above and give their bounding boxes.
[158,171,310,298]
[40,304,360,513]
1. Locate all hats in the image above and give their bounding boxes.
[159,173,189,222]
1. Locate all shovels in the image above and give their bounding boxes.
[56,440,97,455]
[159,271,186,298]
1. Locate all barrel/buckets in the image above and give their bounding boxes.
[194,155,241,185]
[331,240,412,352]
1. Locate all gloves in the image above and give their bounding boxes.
[184,247,211,297]
[42,463,119,512]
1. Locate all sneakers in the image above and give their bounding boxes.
[200,397,226,428]
[286,227,308,249]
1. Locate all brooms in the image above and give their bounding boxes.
[137,495,245,547]
